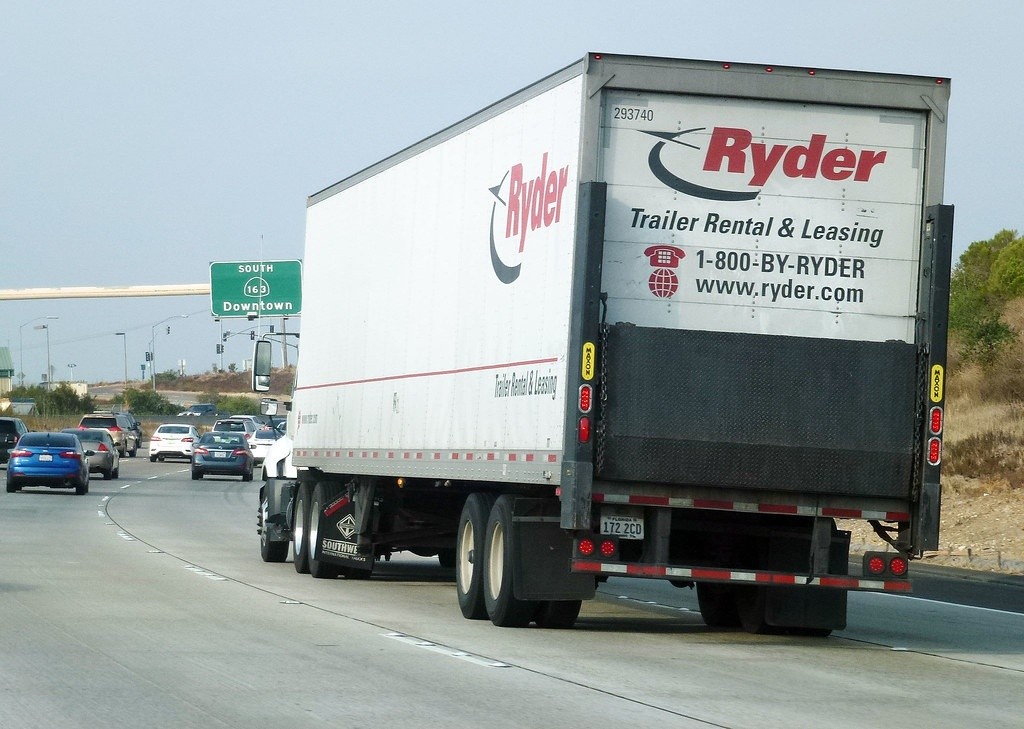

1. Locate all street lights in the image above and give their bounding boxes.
[19,316,58,387]
[151,314,189,391]
[116,331,127,389]
[41,325,51,392]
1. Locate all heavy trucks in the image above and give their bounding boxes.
[248,51,955,637]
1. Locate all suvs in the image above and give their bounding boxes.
[176,404,218,416]
[77,410,143,457]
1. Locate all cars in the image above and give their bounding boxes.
[191,431,258,482]
[5,431,96,494]
[57,427,121,480]
[0,416,36,464]
[148,422,202,462]
[211,413,287,468]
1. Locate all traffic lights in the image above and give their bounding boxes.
[146,352,150,361]
[167,326,171,335]
